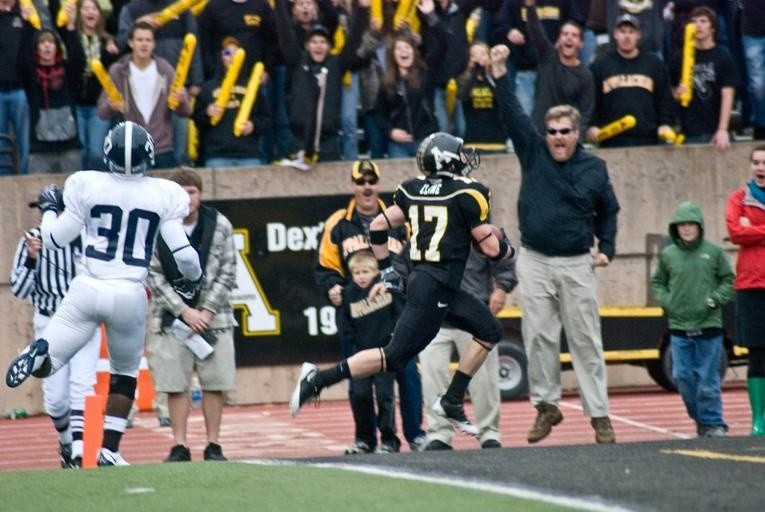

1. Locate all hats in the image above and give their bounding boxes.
[351,160,380,182]
[305,23,331,40]
[614,13,640,30]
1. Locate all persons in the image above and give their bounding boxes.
[0,0,765,177]
[490,45,622,442]
[5,121,237,470]
[726,144,765,436]
[290,133,518,455]
[650,200,736,437]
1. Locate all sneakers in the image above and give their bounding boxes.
[345,395,501,454]
[591,417,615,443]
[7,339,49,388]
[60,443,227,469]
[290,362,319,416]
[698,425,725,440]
[528,401,563,442]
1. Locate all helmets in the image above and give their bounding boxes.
[416,132,480,177]
[102,120,155,180]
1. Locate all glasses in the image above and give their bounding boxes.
[545,128,575,134]
[354,178,377,185]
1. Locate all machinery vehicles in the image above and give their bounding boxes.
[403,224,751,400]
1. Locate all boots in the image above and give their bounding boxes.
[747,377,765,437]
